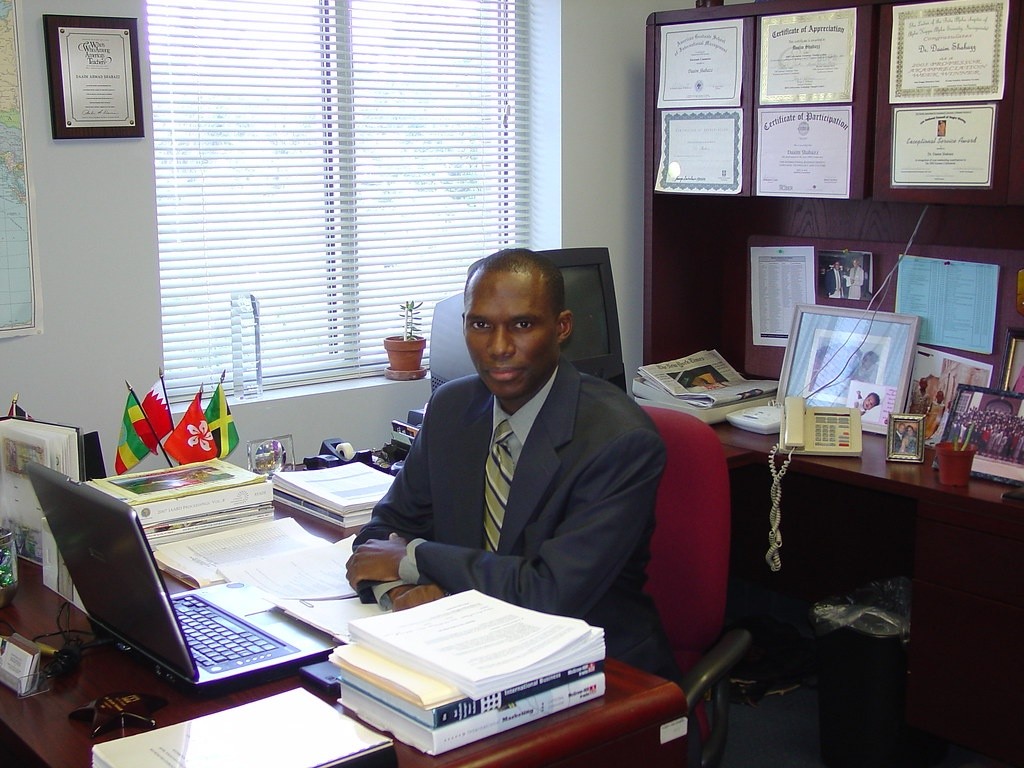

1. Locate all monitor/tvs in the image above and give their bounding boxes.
[430,247,623,394]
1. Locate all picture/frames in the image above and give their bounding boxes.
[932,384,1024,486]
[997,328,1024,394]
[776,304,922,435]
[887,415,925,462]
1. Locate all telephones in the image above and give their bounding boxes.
[778,395,864,456]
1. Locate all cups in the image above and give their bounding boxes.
[935,441,978,485]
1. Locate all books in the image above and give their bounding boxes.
[328,646,605,755]
[92,686,398,768]
[0,417,274,564]
[153,517,335,588]
[270,462,395,528]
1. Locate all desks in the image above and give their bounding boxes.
[0,499,690,768]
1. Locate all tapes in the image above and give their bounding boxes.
[336,442,354,460]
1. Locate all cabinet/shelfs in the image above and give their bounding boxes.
[646,1,1023,767]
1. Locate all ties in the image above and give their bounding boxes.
[482,420,515,552]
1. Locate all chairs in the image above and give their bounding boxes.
[641,404,755,768]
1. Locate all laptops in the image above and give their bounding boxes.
[25,461,346,694]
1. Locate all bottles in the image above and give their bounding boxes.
[0,527,18,609]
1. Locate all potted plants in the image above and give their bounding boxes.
[935,423,980,486]
[382,300,430,380]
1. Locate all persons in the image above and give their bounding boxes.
[809,343,879,396]
[950,408,1024,464]
[824,258,864,300]
[14,526,30,557]
[909,379,953,443]
[346,248,701,768]
[854,390,880,416]
[895,423,916,454]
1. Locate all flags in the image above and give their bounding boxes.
[164,395,217,464]
[204,384,240,460]
[141,381,172,455]
[115,393,153,476]
